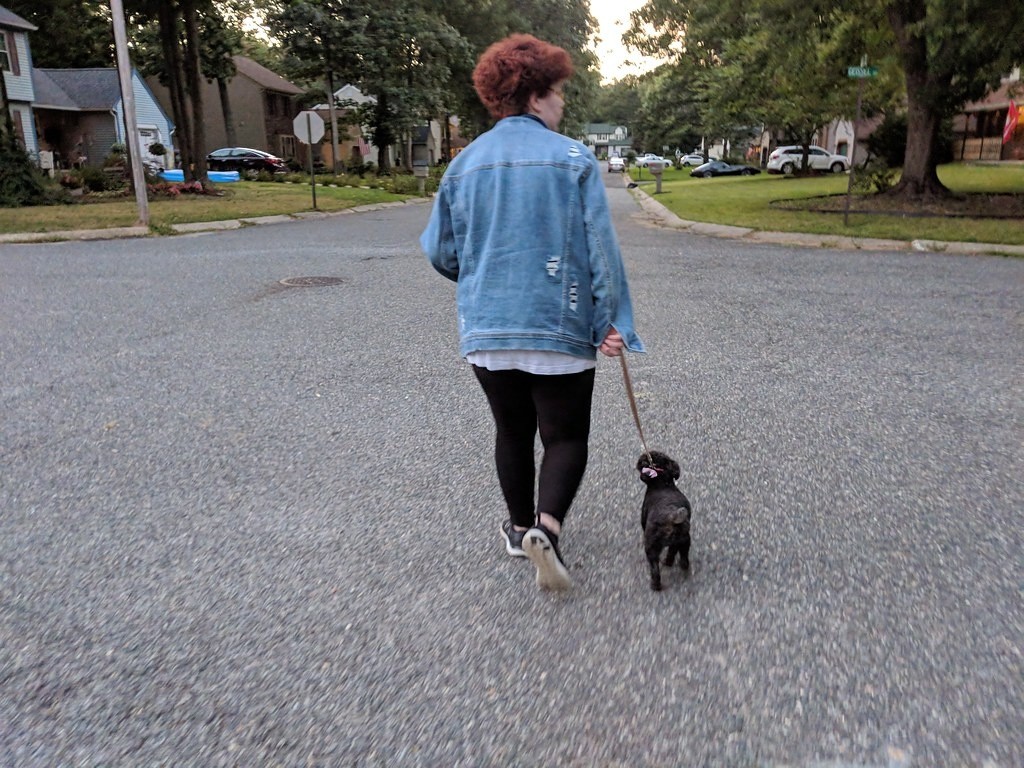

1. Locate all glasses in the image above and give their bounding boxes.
[547,85,564,99]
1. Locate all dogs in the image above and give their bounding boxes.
[634,449,693,590]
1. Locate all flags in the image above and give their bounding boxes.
[1002,98,1020,145]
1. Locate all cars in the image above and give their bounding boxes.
[608,156,626,173]
[679,155,714,167]
[205,148,286,180]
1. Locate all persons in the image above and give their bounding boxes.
[417,32,646,594]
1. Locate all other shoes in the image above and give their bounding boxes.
[522,525,573,592]
[500,518,536,557]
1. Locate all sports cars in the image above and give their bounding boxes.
[690,161,762,178]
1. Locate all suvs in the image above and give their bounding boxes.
[766,146,851,175]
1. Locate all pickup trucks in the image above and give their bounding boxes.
[635,154,672,169]
